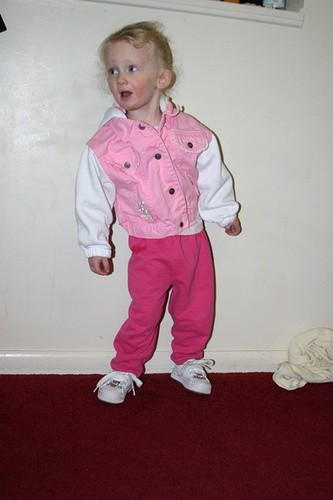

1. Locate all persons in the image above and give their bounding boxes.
[75,21,242,404]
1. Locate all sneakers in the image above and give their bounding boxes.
[170,359,214,394]
[92,370,142,404]
